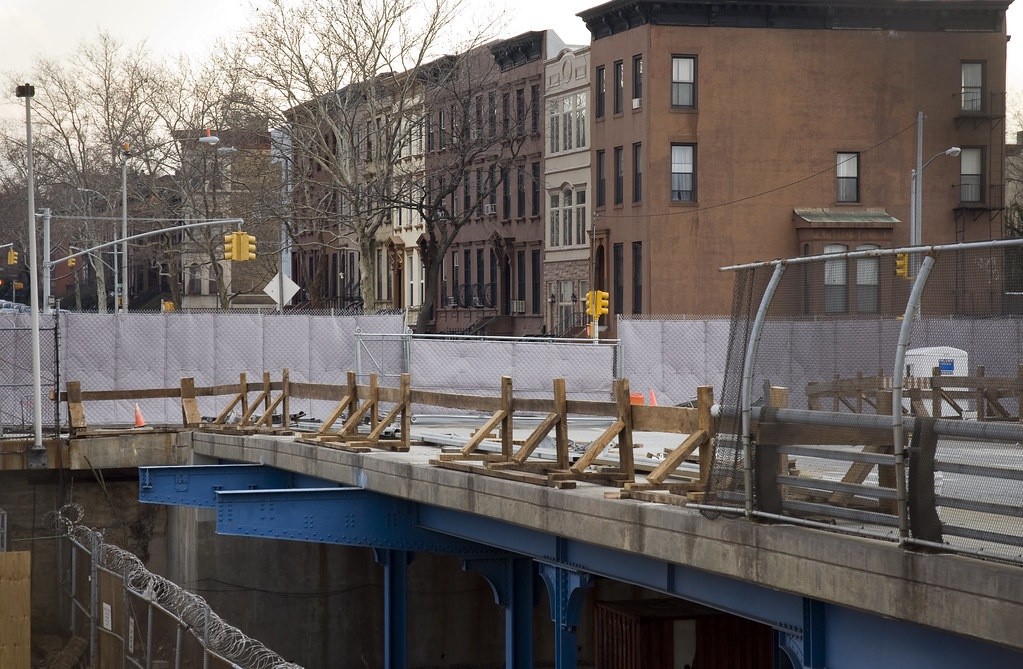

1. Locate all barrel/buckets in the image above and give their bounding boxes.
[630,392,644,405]
[962,410,978,422]
[933,471,943,495]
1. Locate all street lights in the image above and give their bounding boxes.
[218,146,288,314]
[910,147,962,319]
[83,202,118,314]
[121,137,219,313]
[16,84,47,450]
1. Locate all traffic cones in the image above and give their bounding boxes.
[134,403,147,428]
[649,389,658,407]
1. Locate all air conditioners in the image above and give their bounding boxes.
[633,97,642,109]
[510,301,525,313]
[484,204,497,214]
[472,129,482,139]
[448,297,458,306]
[473,297,484,307]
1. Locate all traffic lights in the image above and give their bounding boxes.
[8,250,18,265]
[223,232,239,261]
[895,253,908,280]
[237,231,256,261]
[585,291,597,315]
[68,258,76,267]
[595,290,609,320]
